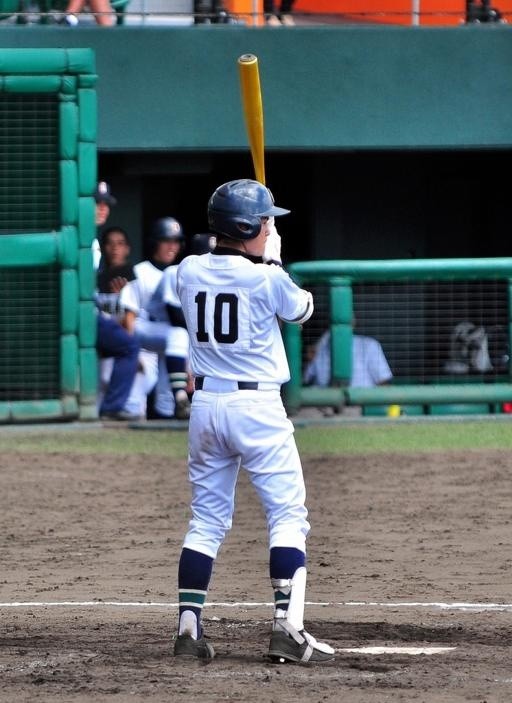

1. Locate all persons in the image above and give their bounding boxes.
[171,181,337,667]
[0,0,298,28]
[464,0,509,25]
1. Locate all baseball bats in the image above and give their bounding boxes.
[238,52,266,185]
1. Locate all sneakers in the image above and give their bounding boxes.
[99,409,140,422]
[267,627,337,664]
[173,633,216,663]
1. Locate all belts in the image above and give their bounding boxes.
[194,376,258,391]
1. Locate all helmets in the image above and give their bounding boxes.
[140,215,184,241]
[206,177,292,238]
[95,181,117,205]
